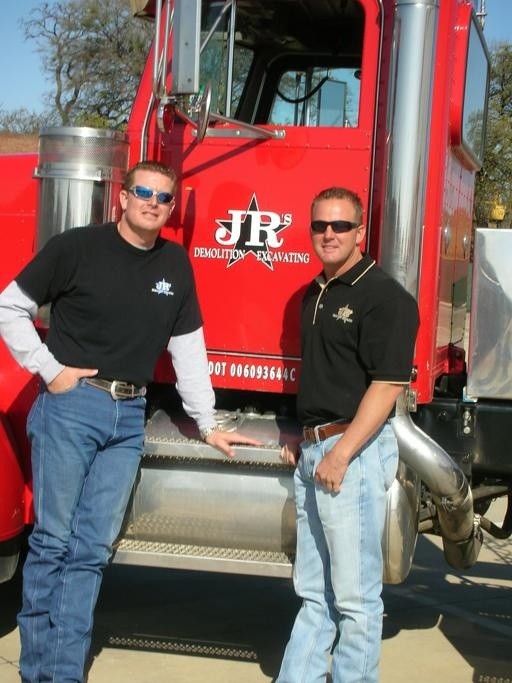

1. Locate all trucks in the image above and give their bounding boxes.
[1,0,510,613]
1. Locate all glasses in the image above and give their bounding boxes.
[121,184,175,207]
[309,220,361,233]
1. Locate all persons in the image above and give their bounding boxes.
[1,157,264,682]
[274,186,420,683]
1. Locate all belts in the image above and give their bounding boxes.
[302,421,352,444]
[82,374,146,401]
